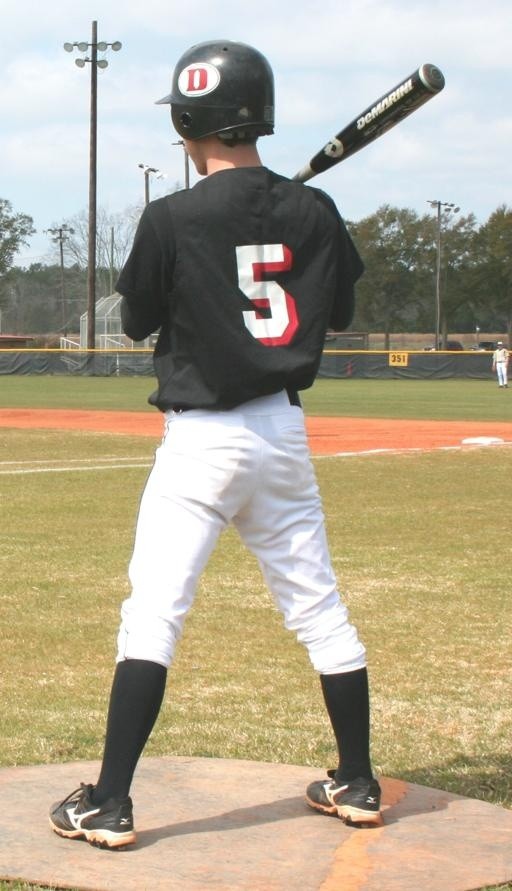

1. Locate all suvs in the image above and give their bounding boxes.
[422,341,463,353]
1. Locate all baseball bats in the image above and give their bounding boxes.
[290,65,445,183]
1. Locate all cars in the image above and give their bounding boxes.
[469,342,508,352]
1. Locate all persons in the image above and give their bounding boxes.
[47,40,382,851]
[491,342,511,388]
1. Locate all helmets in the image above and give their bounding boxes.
[156,40,277,142]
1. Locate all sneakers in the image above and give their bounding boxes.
[49,781,136,847]
[303,768,383,827]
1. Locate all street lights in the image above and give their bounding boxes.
[64,21,122,375]
[170,136,195,190]
[475,319,480,342]
[42,222,76,348]
[135,159,165,209]
[427,198,460,348]
